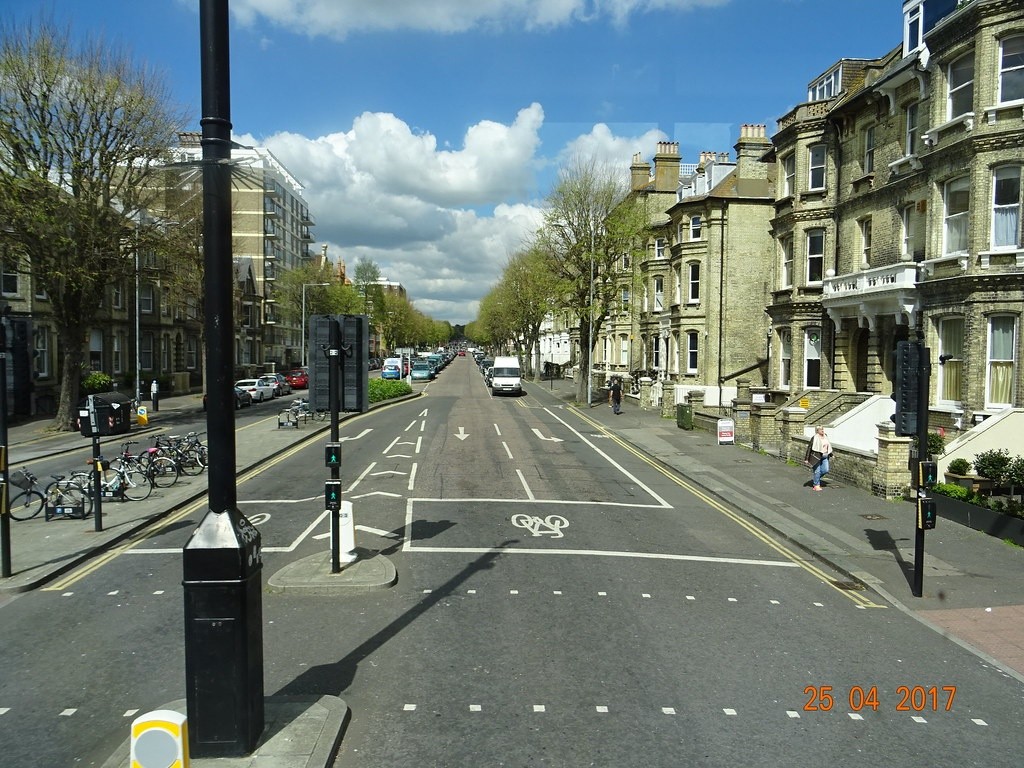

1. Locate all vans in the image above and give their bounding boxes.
[490,355,523,396]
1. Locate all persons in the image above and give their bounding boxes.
[609,379,623,415]
[804,425,834,492]
[544,362,552,377]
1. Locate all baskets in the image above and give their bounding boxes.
[9,472,29,489]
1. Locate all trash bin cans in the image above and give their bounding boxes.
[677,403,692,430]
[76,391,132,437]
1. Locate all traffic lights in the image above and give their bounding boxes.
[325,483,341,511]
[919,461,941,489]
[890,339,916,437]
[325,442,343,469]
[917,497,936,532]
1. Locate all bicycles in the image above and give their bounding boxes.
[66,428,210,499]
[8,466,94,523]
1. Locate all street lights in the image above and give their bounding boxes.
[301,283,330,370]
[132,220,180,410]
[551,221,598,410]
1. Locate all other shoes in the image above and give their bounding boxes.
[813,485,822,491]
[614,409,619,415]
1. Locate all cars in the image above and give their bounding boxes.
[236,379,276,404]
[382,365,400,381]
[457,349,465,357]
[415,360,436,381]
[203,386,253,410]
[368,346,460,373]
[258,373,292,396]
[468,347,494,388]
[286,369,309,389]
